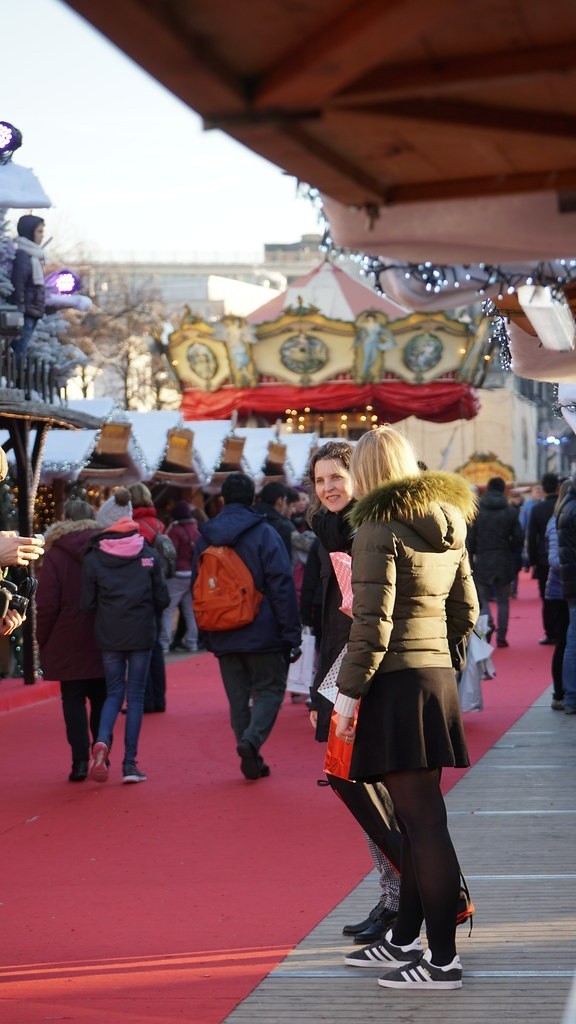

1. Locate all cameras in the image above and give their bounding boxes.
[0,580,29,618]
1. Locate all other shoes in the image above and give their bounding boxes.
[121,706,152,715]
[69,761,88,780]
[538,637,555,645]
[551,699,565,709]
[257,764,270,777]
[237,744,258,779]
[564,706,575,714]
[497,637,508,647]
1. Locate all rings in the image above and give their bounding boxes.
[345,736,348,741]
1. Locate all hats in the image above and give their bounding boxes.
[98,488,133,527]
[172,500,192,520]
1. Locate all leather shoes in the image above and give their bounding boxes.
[343,905,387,936]
[353,909,399,943]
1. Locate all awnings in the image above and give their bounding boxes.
[60,0,575,264]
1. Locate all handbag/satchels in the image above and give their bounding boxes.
[322,696,360,783]
[286,626,316,694]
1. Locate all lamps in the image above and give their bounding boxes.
[517,284,576,350]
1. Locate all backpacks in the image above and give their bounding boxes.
[140,518,177,579]
[192,543,270,632]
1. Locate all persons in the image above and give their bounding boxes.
[0,443,209,784]
[465,472,576,716]
[6,215,47,369]
[191,474,301,780]
[301,441,401,944]
[252,481,315,569]
[333,428,480,988]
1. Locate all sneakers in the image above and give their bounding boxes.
[344,929,423,968]
[378,948,463,989]
[122,767,147,782]
[90,742,109,782]
